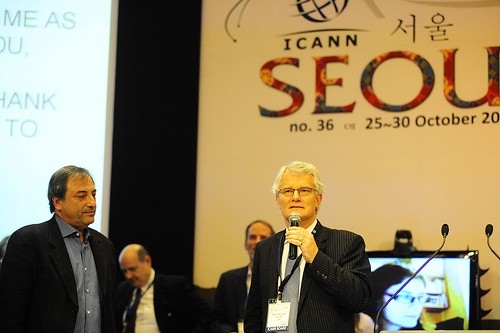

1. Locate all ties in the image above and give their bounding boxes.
[125,280,154,333]
[279,249,301,333]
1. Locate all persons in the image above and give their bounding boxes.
[367,264,428,331]
[351,311,374,333]
[112,244,207,333]
[0,164,117,333]
[243,161,374,333]
[213,220,278,333]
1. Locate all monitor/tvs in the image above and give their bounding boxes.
[365,250,478,331]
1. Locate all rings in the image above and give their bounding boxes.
[299,242,304,247]
[299,236,304,241]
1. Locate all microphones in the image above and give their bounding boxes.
[372,223,449,332]
[289,212,301,259]
[485,224,500,260]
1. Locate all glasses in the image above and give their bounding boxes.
[386,292,427,306]
[277,186,317,198]
